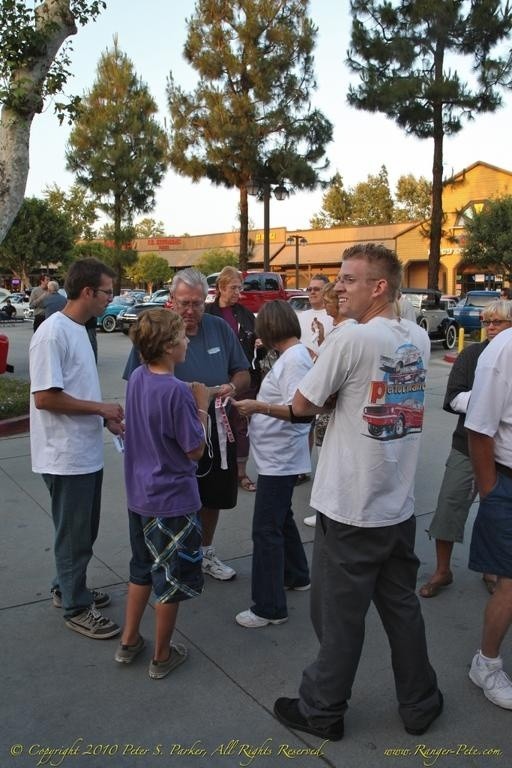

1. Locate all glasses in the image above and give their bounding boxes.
[482,319,512,327]
[334,275,379,284]
[322,298,336,306]
[173,293,206,311]
[91,287,114,298]
[231,286,244,293]
[307,287,321,292]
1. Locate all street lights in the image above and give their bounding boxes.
[283,236,310,290]
[244,176,288,272]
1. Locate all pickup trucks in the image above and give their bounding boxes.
[205,271,310,311]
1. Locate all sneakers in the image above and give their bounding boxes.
[303,514,316,529]
[482,573,499,597]
[274,697,344,741]
[149,641,188,678]
[468,650,512,709]
[114,632,148,664]
[236,608,289,628]
[65,601,121,639]
[419,571,453,599]
[282,583,311,591]
[201,548,237,582]
[51,587,111,609]
[405,689,443,736]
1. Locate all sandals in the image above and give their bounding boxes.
[237,473,257,492]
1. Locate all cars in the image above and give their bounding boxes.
[96,292,150,332]
[379,347,424,372]
[118,287,179,336]
[399,287,461,349]
[361,398,423,437]
[0,293,35,320]
[448,289,512,333]
[390,369,425,384]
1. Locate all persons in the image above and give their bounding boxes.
[461,323,512,712]
[0,300,17,320]
[25,263,358,680]
[417,296,512,597]
[273,243,444,740]
